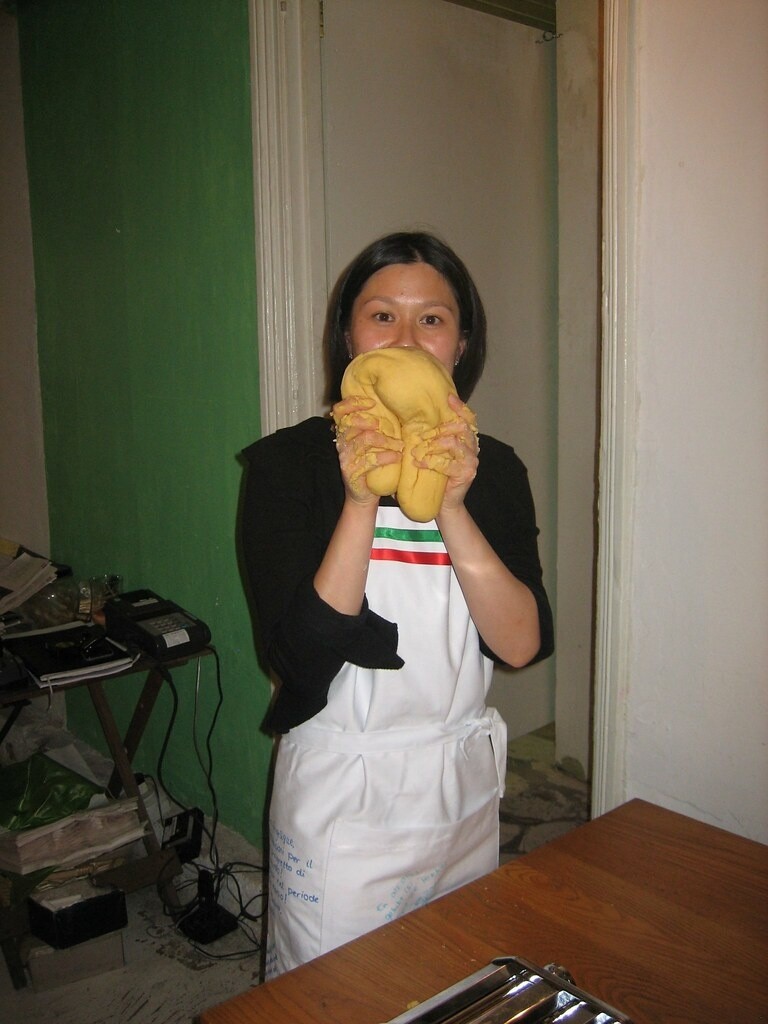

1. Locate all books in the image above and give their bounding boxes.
[12,625,133,689]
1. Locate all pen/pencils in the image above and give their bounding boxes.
[104,634,127,652]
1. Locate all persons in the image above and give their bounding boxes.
[241,232,555,983]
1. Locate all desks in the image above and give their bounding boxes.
[0,643,214,992]
[190,797,768,1024]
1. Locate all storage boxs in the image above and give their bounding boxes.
[22,928,128,990]
[0,796,146,875]
[30,880,128,950]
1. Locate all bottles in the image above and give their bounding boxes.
[19,563,80,625]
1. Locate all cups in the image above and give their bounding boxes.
[89,574,123,611]
